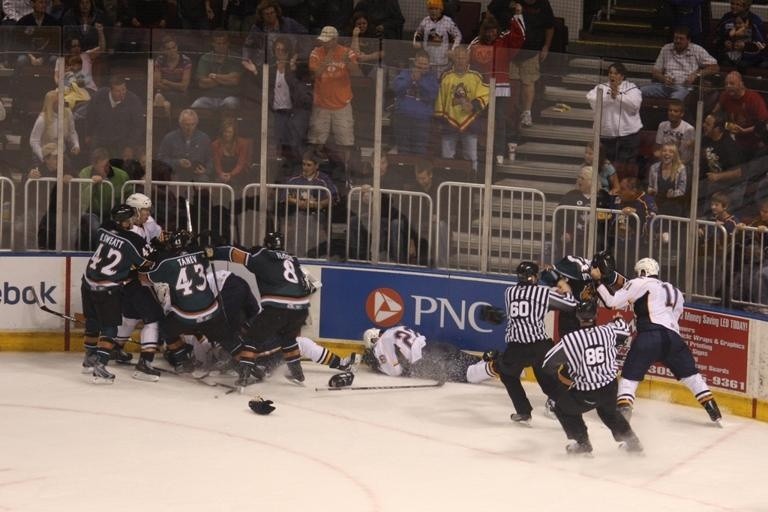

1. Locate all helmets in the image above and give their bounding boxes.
[111,203,135,230]
[363,327,383,350]
[633,257,660,278]
[590,249,616,283]
[126,192,152,209]
[575,299,597,322]
[516,261,540,286]
[264,231,284,250]
[169,227,194,255]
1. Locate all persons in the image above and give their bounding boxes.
[553,1,768,312]
[591,257,722,423]
[497,260,577,421]
[113,192,165,376]
[539,300,642,453]
[80,204,159,380]
[540,249,629,411]
[362,324,526,384]
[1,2,558,265]
[147,230,357,381]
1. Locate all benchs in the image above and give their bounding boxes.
[0,0,768,305]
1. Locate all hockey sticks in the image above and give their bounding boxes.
[32,283,141,346]
[118,360,239,398]
[313,373,448,391]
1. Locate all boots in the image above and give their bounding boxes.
[704,400,722,421]
[186,345,203,367]
[619,437,643,451]
[235,366,250,387]
[107,347,133,360]
[83,352,96,367]
[545,398,556,411]
[511,412,531,421]
[174,358,210,378]
[565,439,593,455]
[618,401,633,422]
[92,361,115,379]
[135,355,161,377]
[285,364,305,382]
[329,352,355,371]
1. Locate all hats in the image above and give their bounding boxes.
[316,26,338,43]
[426,1,444,11]
